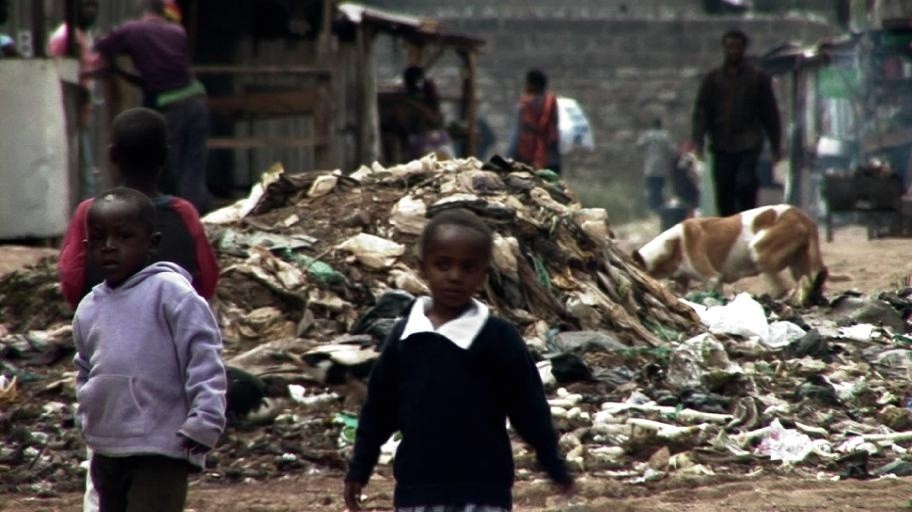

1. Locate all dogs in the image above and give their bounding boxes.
[631,203,852,300]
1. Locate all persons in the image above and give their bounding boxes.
[97,2,217,218]
[510,67,561,179]
[58,107,220,510]
[345,208,575,512]
[390,64,454,160]
[71,188,229,511]
[447,97,496,163]
[693,31,784,216]
[638,116,677,211]
[49,1,97,200]
